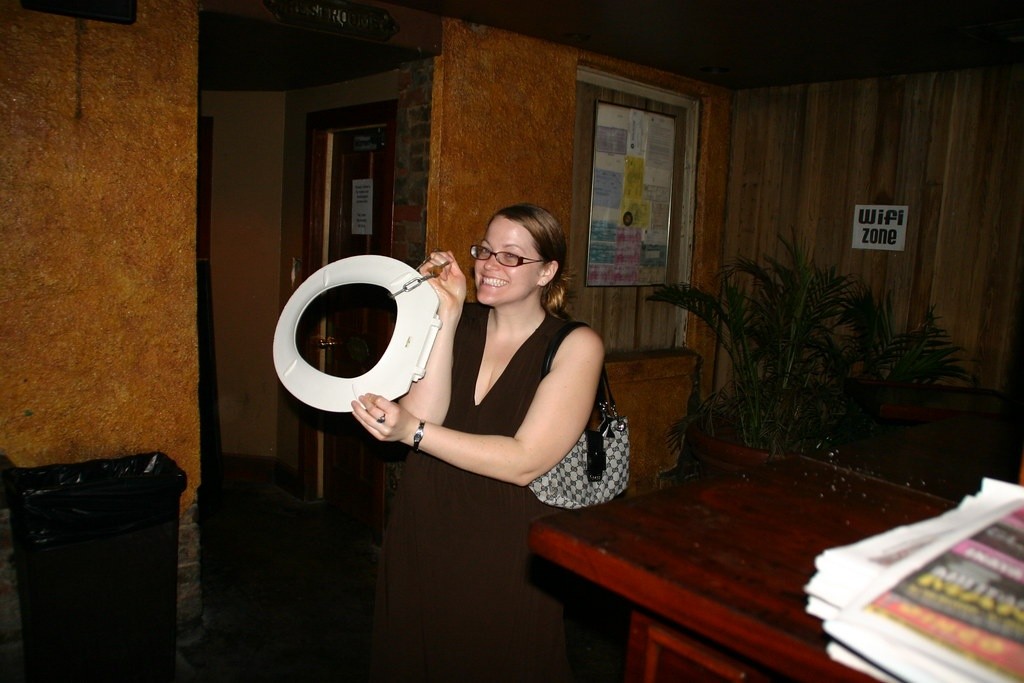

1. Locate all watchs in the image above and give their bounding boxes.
[413,419,426,451]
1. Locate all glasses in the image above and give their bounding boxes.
[470,245,543,267]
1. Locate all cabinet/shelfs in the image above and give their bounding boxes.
[525,451,1024,683]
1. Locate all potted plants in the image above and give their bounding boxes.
[644,223,973,474]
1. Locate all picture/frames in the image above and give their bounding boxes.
[583,95,675,286]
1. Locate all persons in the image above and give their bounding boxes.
[350,204,606,683]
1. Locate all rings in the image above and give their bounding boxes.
[377,413,385,423]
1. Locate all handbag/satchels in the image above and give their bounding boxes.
[528,322,631,508]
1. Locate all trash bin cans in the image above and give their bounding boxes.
[8,451,189,683]
